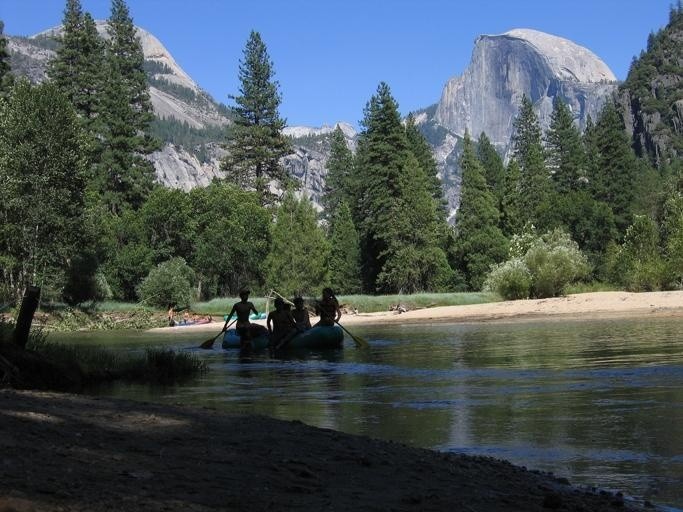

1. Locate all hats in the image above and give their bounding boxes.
[240,288,250,297]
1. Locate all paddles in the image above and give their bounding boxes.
[199,306,251,348]
[316,304,366,347]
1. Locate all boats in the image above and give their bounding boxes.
[270,323,343,354]
[222,324,268,348]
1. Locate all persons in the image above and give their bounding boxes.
[183,310,189,323]
[283,303,295,328]
[266,297,292,355]
[351,307,357,316]
[167,306,173,322]
[191,312,212,321]
[288,297,312,330]
[311,287,341,329]
[339,303,348,314]
[221,288,258,356]
